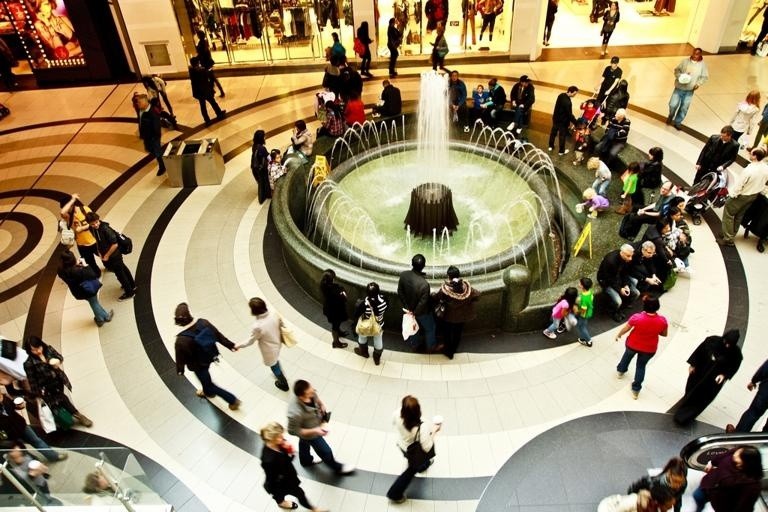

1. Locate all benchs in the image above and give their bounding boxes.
[529,118,677,333]
[297,98,533,172]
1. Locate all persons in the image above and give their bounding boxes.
[189,32,227,123]
[261,422,314,510]
[597,445,764,512]
[424,0,503,49]
[317,18,402,136]
[547,57,632,166]
[692,90,768,246]
[235,297,290,392]
[175,303,240,412]
[250,120,312,204]
[448,71,536,135]
[652,1,676,16]
[542,221,675,400]
[320,268,387,365]
[387,395,440,504]
[57,193,135,328]
[752,6,768,56]
[581,147,695,272]
[590,1,620,56]
[667,48,708,130]
[674,329,768,434]
[431,26,452,77]
[543,1,558,45]
[398,254,481,359]
[134,74,178,176]
[0,335,93,504]
[84,473,130,505]
[287,379,356,475]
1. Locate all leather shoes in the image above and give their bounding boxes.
[278,502,299,510]
[410,343,454,360]
[386,495,406,503]
[725,424,735,433]
[275,381,289,392]
[312,455,323,464]
[95,309,114,326]
[341,464,357,473]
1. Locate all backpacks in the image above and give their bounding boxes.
[100,221,132,255]
[177,318,222,370]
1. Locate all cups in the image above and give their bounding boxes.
[28,459,42,470]
[433,415,443,424]
[49,358,58,369]
[13,396,24,407]
[321,422,329,432]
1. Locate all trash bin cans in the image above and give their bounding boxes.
[161,137,227,188]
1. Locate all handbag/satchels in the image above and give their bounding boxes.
[50,403,74,430]
[78,266,103,295]
[34,397,58,434]
[355,300,381,337]
[353,36,366,56]
[400,426,436,474]
[280,322,294,346]
[435,39,449,57]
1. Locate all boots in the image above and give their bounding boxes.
[72,411,93,428]
[332,327,348,348]
[354,341,370,359]
[373,347,383,365]
[600,42,610,57]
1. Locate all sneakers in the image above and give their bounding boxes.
[577,337,592,347]
[506,122,523,134]
[674,123,685,130]
[632,391,640,401]
[118,292,136,302]
[715,232,734,246]
[618,371,626,380]
[666,115,673,124]
[557,324,566,334]
[204,110,226,127]
[543,329,557,339]
[608,310,626,322]
[463,125,470,133]
[228,398,241,411]
[548,144,570,156]
[196,389,216,399]
